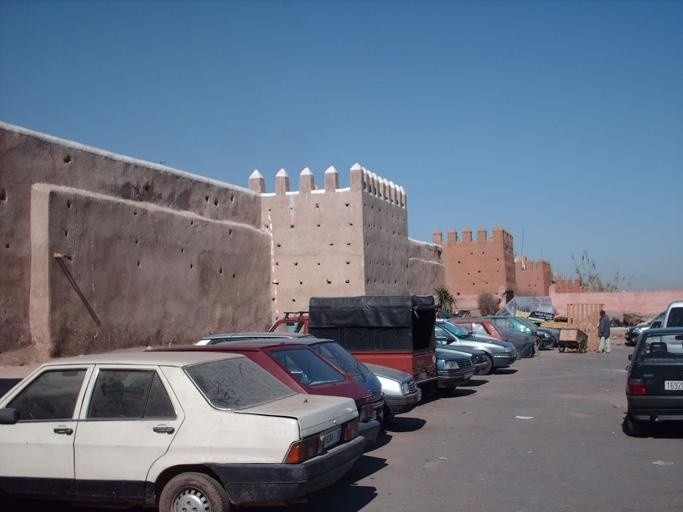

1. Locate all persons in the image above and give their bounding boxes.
[595,310,611,353]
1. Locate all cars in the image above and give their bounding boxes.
[599,300,683,355]
[0,351,364,511]
[624,326,683,436]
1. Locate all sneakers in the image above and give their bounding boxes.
[597,350,611,353]
